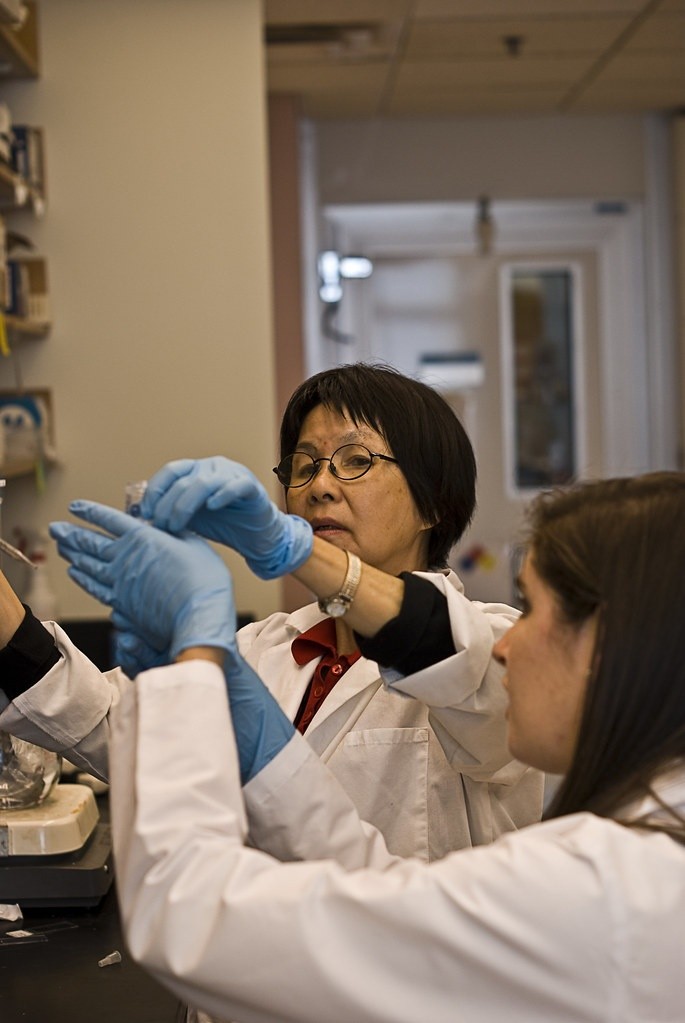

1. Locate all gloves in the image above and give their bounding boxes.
[46,497,244,676]
[141,455,312,581]
[107,607,295,791]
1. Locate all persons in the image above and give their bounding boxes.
[50,464,683,1023]
[0,361,547,1023]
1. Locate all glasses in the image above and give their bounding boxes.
[273,443,399,489]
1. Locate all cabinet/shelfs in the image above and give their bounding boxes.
[0,0,61,487]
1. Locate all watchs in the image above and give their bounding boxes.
[317,548,363,618]
